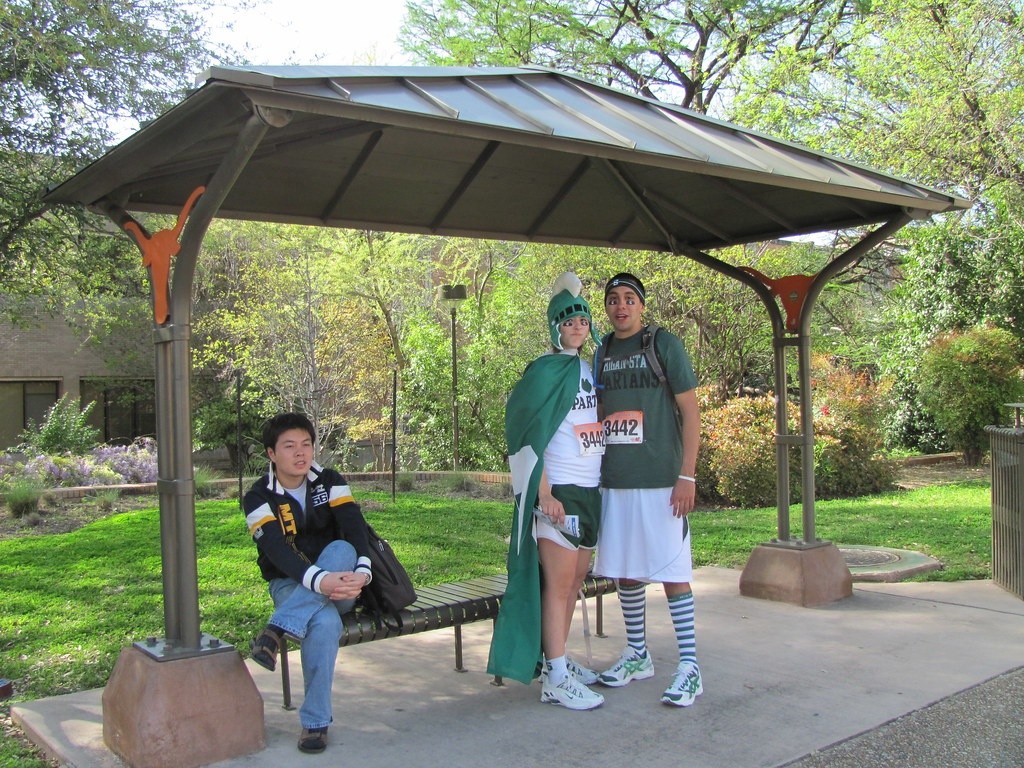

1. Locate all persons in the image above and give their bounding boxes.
[505,272,604,710]
[244,413,371,754]
[592,273,703,706]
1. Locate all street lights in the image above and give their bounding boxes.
[438,283,469,472]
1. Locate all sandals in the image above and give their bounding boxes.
[298,726,329,753]
[249,629,281,671]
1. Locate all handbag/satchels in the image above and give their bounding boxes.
[337,516,418,632]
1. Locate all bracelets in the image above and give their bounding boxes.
[362,572,369,584]
[678,475,695,482]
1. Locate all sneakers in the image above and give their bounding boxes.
[537,652,600,685]
[540,671,605,710]
[660,663,703,706]
[597,646,655,686]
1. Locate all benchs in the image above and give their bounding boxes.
[279,572,616,712]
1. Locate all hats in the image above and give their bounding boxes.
[546,272,603,351]
